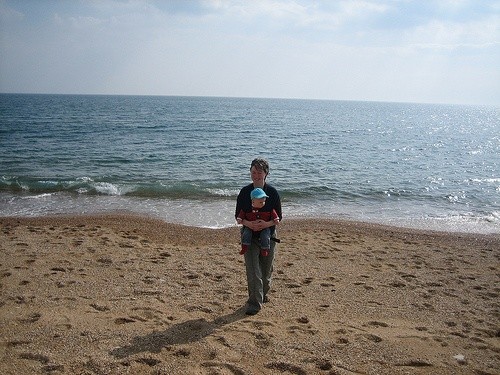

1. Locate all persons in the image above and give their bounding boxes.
[235,156,283,313]
[237,187,280,256]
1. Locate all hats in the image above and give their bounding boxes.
[250,188,268,200]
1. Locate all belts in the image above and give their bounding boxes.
[269,237,280,243]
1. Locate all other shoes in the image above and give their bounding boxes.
[262,295,269,302]
[247,309,255,315]
